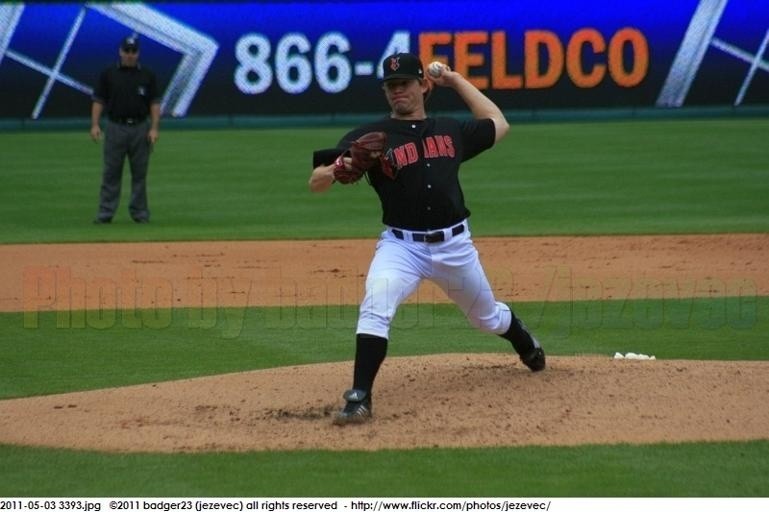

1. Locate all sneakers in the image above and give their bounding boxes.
[514,317,545,371]
[341,390,372,424]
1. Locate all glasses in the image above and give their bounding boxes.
[123,47,138,54]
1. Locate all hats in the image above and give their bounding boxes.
[121,37,139,49]
[382,53,424,83]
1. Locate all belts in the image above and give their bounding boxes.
[116,118,145,126]
[391,225,464,244]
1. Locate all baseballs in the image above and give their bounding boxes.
[428,61,443,77]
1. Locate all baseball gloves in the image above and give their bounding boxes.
[332,131,390,185]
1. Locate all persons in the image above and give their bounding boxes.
[307,54,545,419]
[91,34,162,221]
